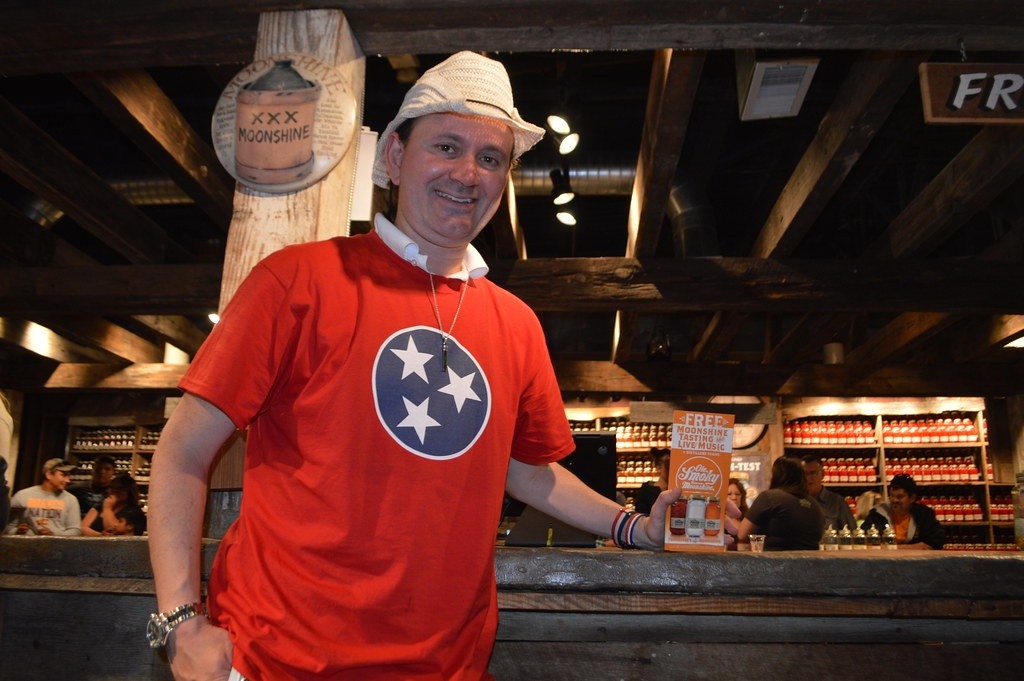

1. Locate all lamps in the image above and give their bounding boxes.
[544,98,577,134]
[550,162,577,206]
[555,197,586,226]
[542,122,583,157]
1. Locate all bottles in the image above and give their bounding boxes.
[686,493,706,538]
[73,428,162,513]
[704,497,721,536]
[782,418,1015,522]
[669,495,687,535]
[569,418,673,505]
[818,523,898,551]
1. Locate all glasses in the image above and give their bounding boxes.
[894,473,912,479]
[804,470,822,477]
[51,460,70,470]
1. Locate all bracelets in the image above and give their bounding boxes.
[611,506,646,548]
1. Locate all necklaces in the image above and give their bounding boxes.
[428,265,468,371]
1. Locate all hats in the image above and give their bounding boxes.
[370,50,545,190]
[43,459,77,475]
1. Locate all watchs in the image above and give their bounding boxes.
[146,602,207,648]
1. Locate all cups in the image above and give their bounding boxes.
[748,534,766,554]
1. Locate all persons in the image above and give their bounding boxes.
[146,49,742,681]
[633,448,670,517]
[800,456,843,530]
[72,456,115,518]
[724,477,749,551]
[852,491,881,519]
[859,472,946,550]
[5,458,81,536]
[737,455,825,551]
[81,474,145,537]
[0,455,10,531]
[102,502,145,537]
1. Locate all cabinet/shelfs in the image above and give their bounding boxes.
[59,413,167,507]
[567,405,673,492]
[776,400,1015,549]
